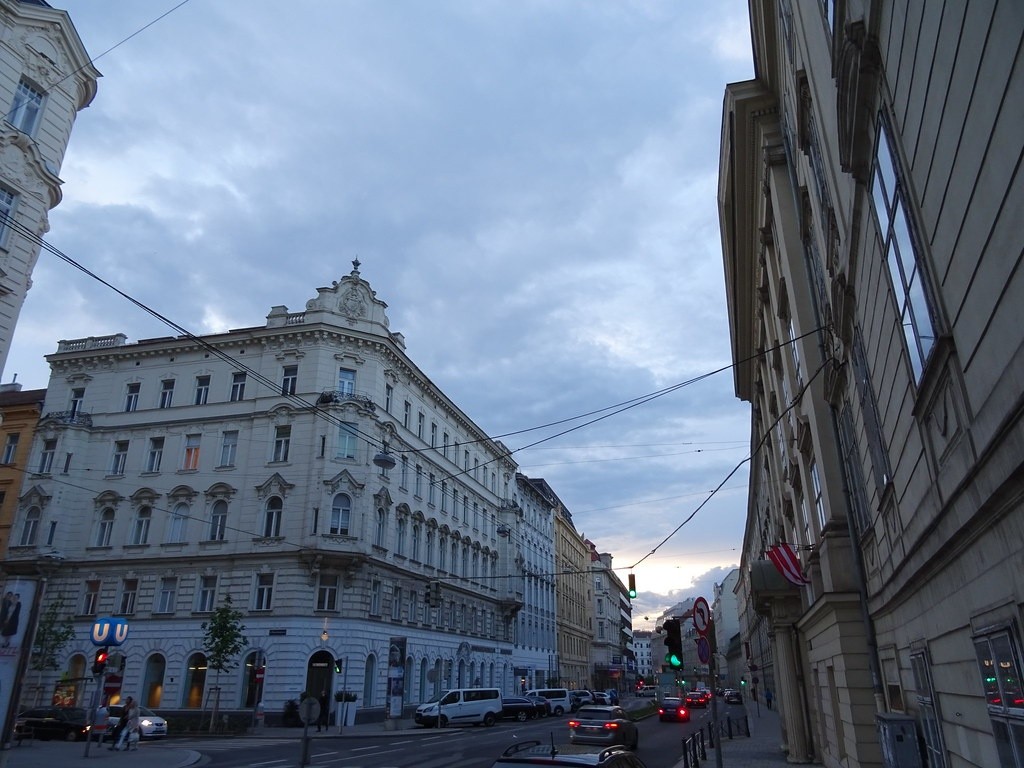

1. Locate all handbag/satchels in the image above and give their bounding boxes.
[127,732,139,742]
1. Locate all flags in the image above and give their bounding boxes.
[766,544,811,589]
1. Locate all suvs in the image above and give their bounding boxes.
[569,705,638,747]
[488,740,648,768]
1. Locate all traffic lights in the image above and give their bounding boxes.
[663,619,683,669]
[741,680,746,685]
[335,658,341,673]
[682,677,684,683]
[94,648,108,673]
[629,575,636,597]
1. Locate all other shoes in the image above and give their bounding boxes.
[130,748,137,751]
[106,747,114,751]
[114,747,120,751]
[123,748,130,751]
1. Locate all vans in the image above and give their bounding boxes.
[415,688,503,727]
[521,688,571,717]
[94,704,167,742]
[636,685,658,697]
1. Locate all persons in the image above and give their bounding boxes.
[766,688,773,710]
[0,591,21,648]
[316,690,329,732]
[583,685,588,690]
[113,699,141,751]
[610,688,619,706]
[106,696,132,751]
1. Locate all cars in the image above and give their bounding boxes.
[499,697,550,722]
[716,687,741,704]
[660,697,690,721]
[686,687,711,708]
[569,690,611,705]
[16,705,90,741]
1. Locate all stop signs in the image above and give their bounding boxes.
[255,668,265,681]
